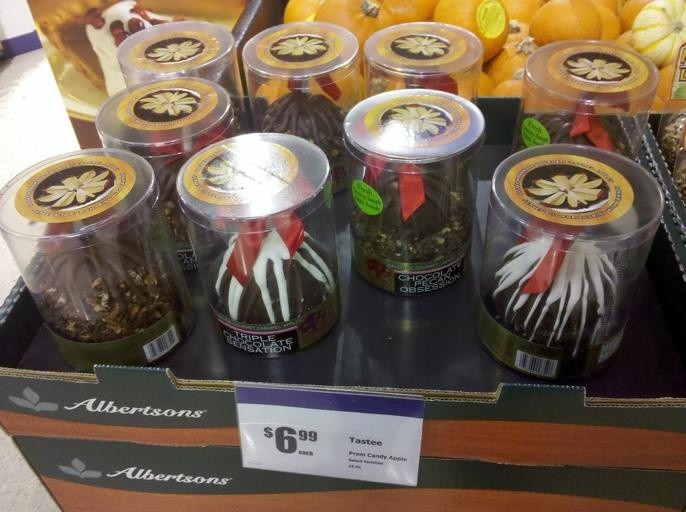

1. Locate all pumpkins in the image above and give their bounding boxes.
[254,1,686,114]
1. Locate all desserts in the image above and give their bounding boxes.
[29,88,686,346]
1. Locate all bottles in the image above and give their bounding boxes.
[516,38,660,163]
[338,88,489,294]
[96,75,240,277]
[117,20,249,133]
[173,129,344,360]
[241,20,362,197]
[363,21,484,106]
[478,141,665,384]
[2,147,198,366]
